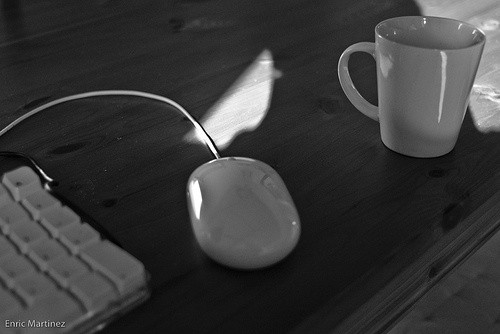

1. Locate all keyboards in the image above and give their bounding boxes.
[1,165,150,333]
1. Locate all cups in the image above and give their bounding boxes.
[336,15,487,159]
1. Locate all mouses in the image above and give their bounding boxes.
[187,156,302,271]
